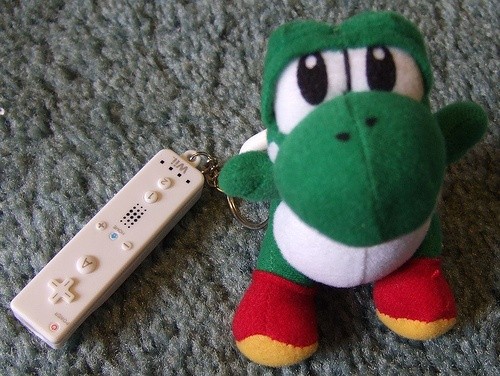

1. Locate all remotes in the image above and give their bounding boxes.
[10,147,206,350]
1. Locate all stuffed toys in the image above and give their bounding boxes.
[217,8,489,367]
